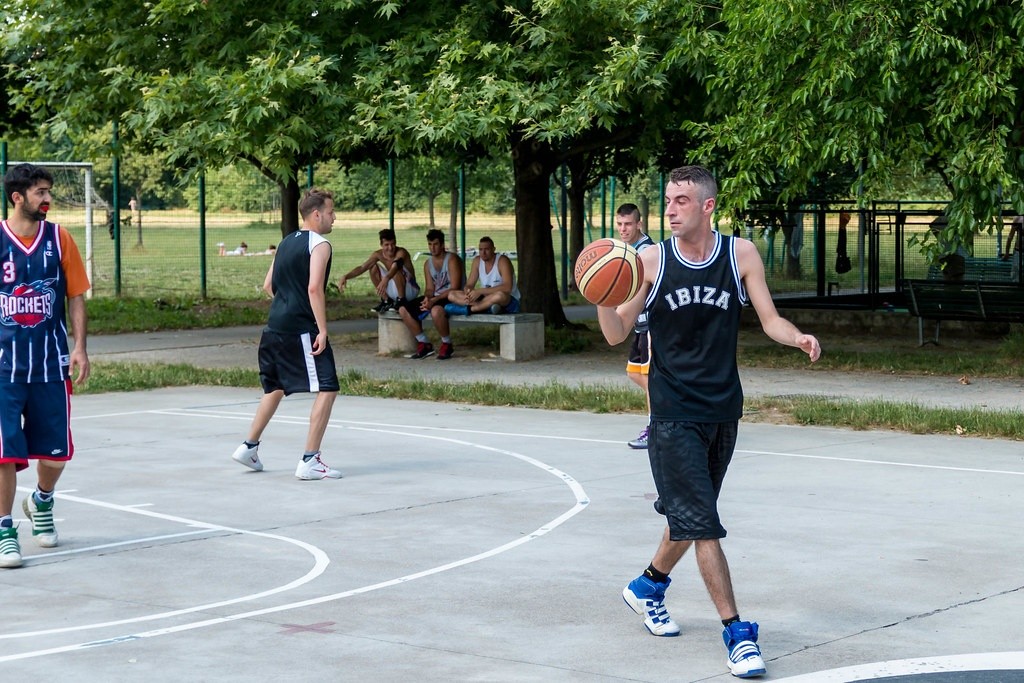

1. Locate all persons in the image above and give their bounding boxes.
[218,242,250,256]
[233,189,343,480]
[0,163,91,568]
[338,229,420,312]
[614,203,656,449]
[128,197,137,217]
[596,165,822,678]
[444,237,520,314]
[250,245,277,256]
[398,229,466,360]
[1000,215,1024,283]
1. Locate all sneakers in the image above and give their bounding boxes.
[490,303,501,315]
[388,295,409,313]
[411,341,434,361]
[293,452,344,481]
[443,302,472,316]
[622,573,683,637]
[722,621,768,678]
[370,295,393,315]
[436,341,455,359]
[628,425,651,450]
[0,523,23,567]
[22,492,59,548]
[232,443,265,473]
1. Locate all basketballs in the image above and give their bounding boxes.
[574,238,644,307]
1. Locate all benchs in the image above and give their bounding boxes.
[900,279,1024,346]
[378,313,545,361]
[926,258,1013,282]
